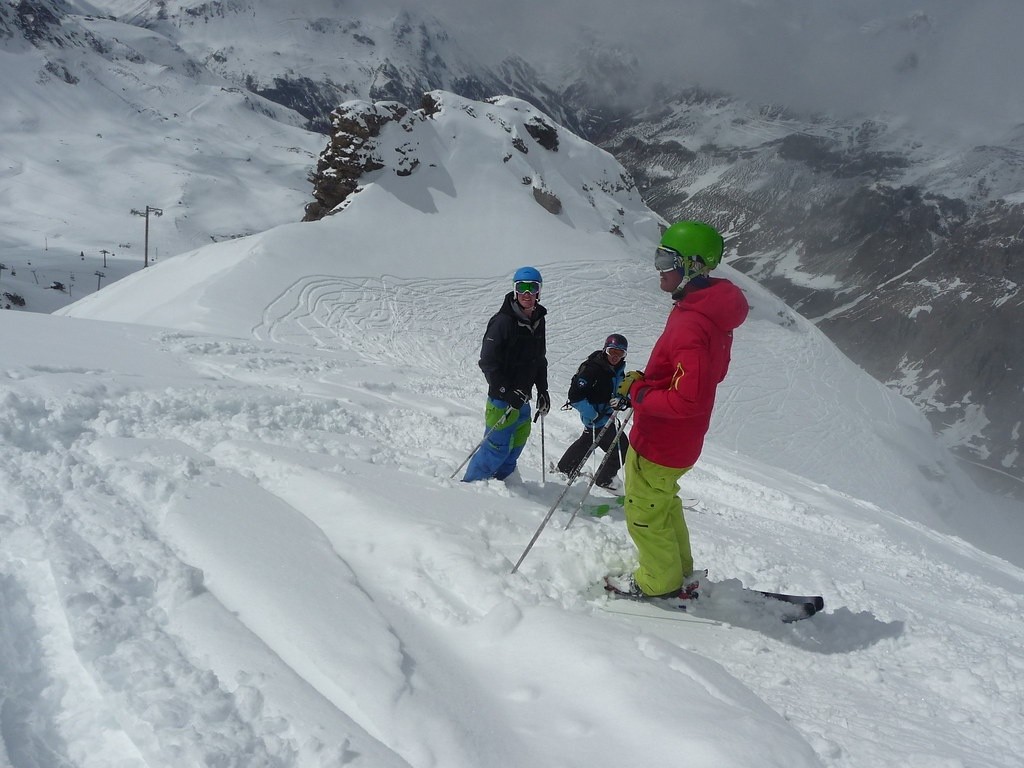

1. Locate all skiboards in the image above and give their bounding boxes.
[600,574,824,624]
[547,462,700,509]
[555,495,626,518]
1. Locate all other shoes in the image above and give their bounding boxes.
[607,572,682,601]
[599,479,616,491]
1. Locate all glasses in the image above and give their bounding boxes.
[606,347,627,358]
[514,281,541,295]
[654,246,679,273]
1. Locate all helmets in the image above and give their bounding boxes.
[660,222,723,270]
[513,267,542,283]
[604,334,627,351]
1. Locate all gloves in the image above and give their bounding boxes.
[593,413,609,427]
[492,379,527,409]
[617,369,646,398]
[535,378,550,415]
[610,392,626,410]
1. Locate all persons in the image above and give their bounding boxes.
[463,267,551,483]
[558,335,630,485]
[603,221,749,597]
